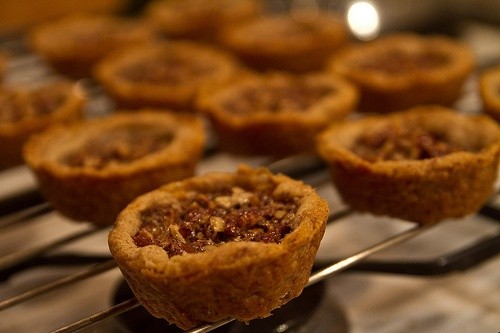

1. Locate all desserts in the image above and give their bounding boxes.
[0,0,499,331]
